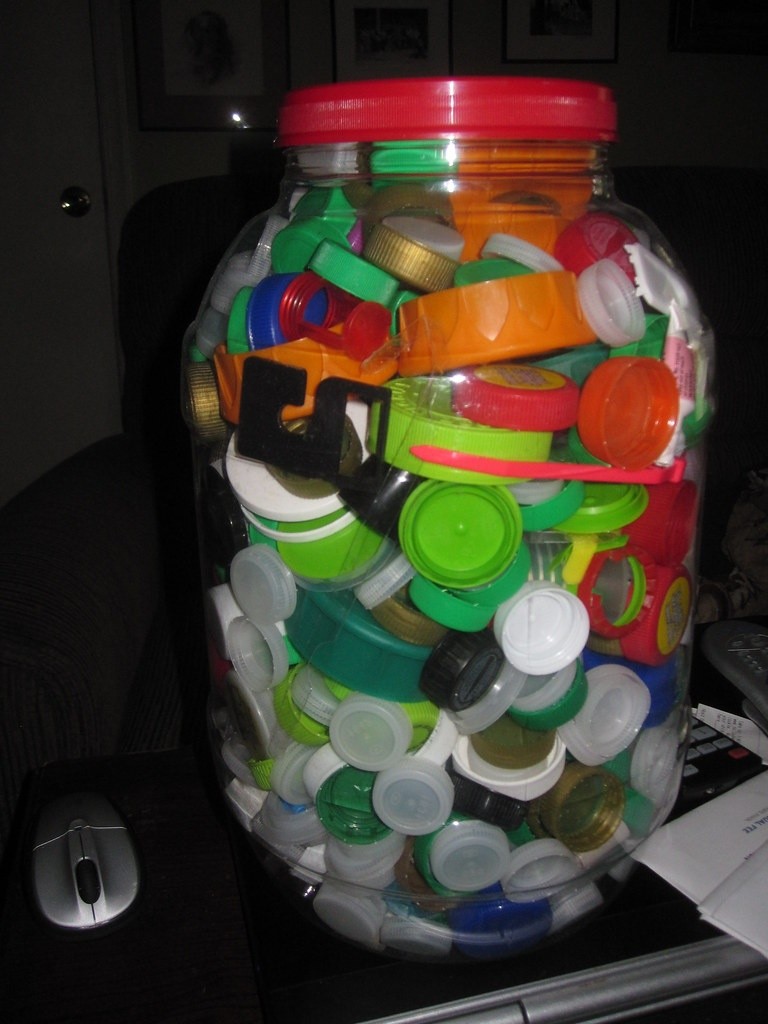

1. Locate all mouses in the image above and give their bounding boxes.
[32,794,141,930]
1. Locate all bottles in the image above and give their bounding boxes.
[186,74,714,950]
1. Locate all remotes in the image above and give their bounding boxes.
[698,620,768,722]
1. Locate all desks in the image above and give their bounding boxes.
[1,748,767,1024]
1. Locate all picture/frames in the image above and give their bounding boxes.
[131,0,291,132]
[501,0,619,64]
[331,0,454,82]
[668,0,768,56]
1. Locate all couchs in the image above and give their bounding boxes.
[1,164,767,856]
